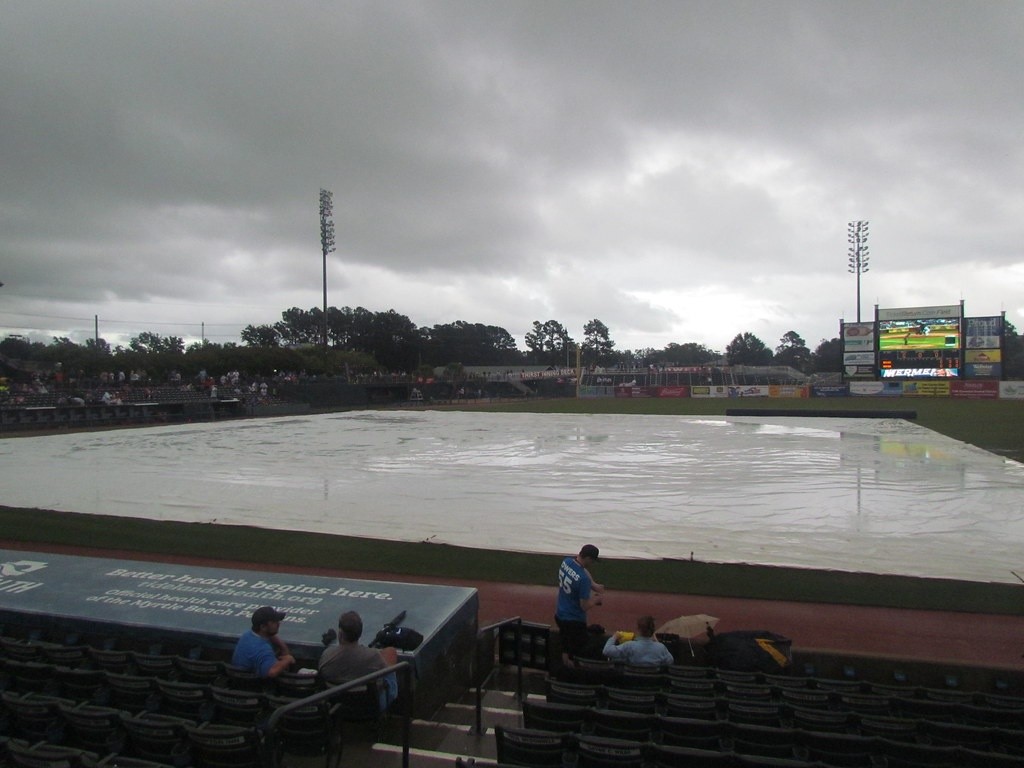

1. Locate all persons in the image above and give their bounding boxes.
[317,610,397,717]
[879,319,956,345]
[0,362,712,407]
[232,606,295,697]
[555,544,605,647]
[546,615,674,682]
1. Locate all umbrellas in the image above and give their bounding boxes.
[655,614,720,657]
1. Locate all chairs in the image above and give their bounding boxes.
[454,628,1024,768]
[0,617,402,768]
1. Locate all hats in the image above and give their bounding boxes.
[252,606,286,624]
[582,545,602,562]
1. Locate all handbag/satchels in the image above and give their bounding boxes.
[377,623,423,651]
